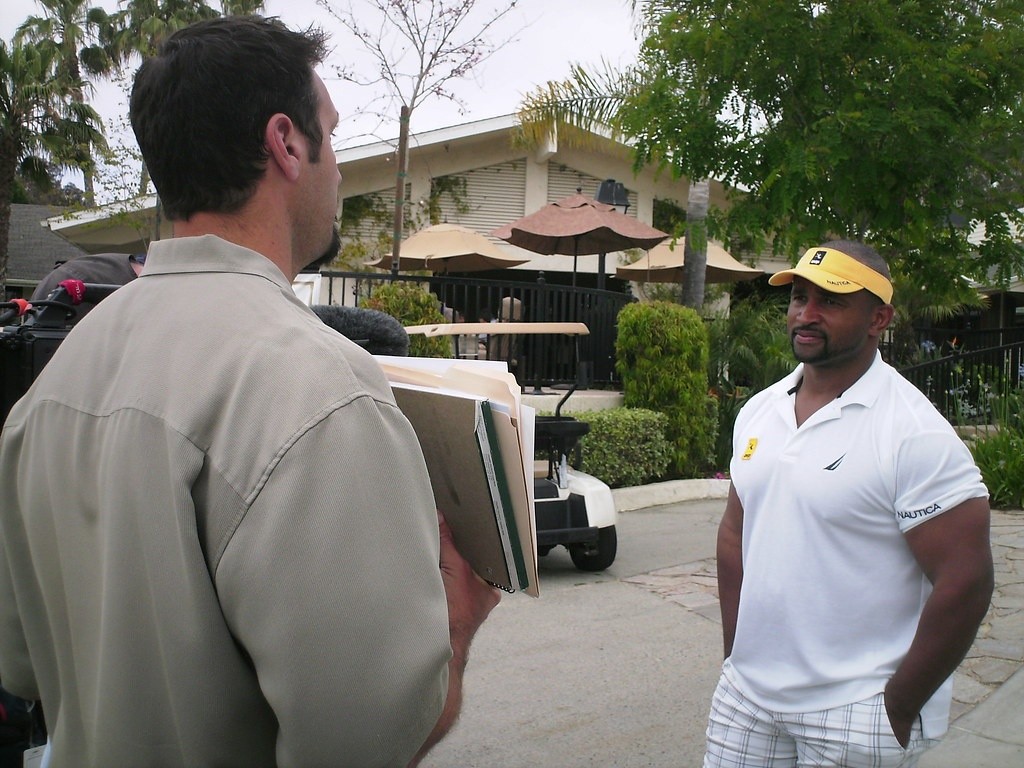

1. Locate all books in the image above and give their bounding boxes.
[369,380,530,598]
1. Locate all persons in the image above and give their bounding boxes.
[0,11,504,766]
[941,335,960,395]
[459,314,502,350]
[702,238,996,768]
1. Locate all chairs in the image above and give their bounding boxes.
[534,416,590,479]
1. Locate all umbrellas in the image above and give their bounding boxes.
[488,185,672,287]
[615,235,766,284]
[362,214,532,317]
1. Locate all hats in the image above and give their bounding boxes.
[768,246,894,306]
[26,253,139,389]
